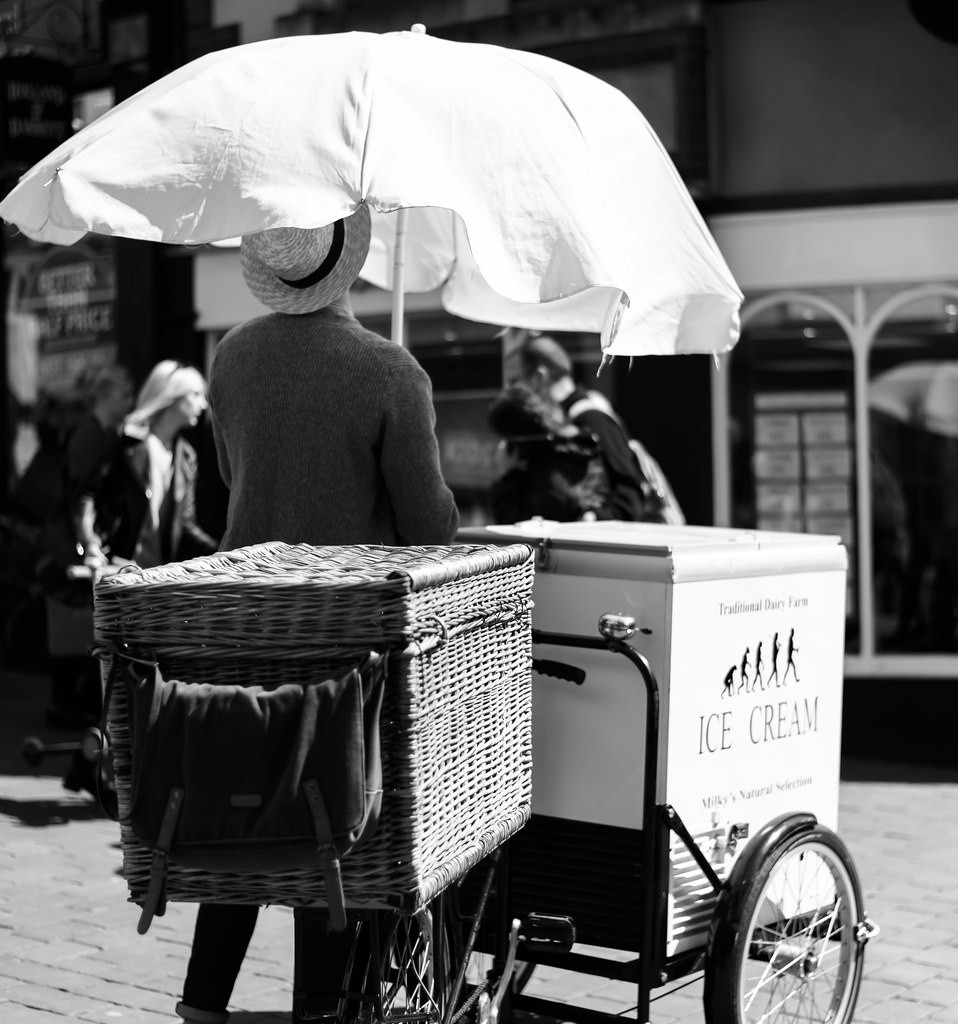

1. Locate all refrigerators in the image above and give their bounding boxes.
[453,516,848,959]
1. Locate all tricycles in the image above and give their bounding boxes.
[155,518,877,1024]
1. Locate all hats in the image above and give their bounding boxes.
[240,200,371,315]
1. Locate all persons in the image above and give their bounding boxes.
[15,359,218,811]
[177,203,462,1024]
[471,336,667,525]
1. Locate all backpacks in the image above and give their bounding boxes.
[568,386,686,524]
[15,418,111,527]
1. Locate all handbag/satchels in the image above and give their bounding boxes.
[126,650,385,932]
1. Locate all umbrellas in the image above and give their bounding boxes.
[1,23,742,377]
[870,362,957,439]
